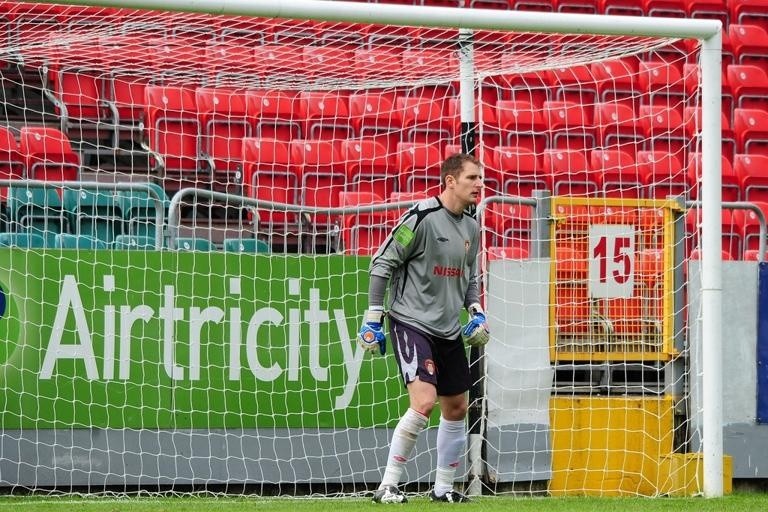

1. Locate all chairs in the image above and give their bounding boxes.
[0,0,767,340]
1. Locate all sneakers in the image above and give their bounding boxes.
[429,489,472,504]
[371,485,408,504]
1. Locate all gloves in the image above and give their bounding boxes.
[463,312,490,347]
[357,309,387,355]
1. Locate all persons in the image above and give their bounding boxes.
[356,153,490,504]
